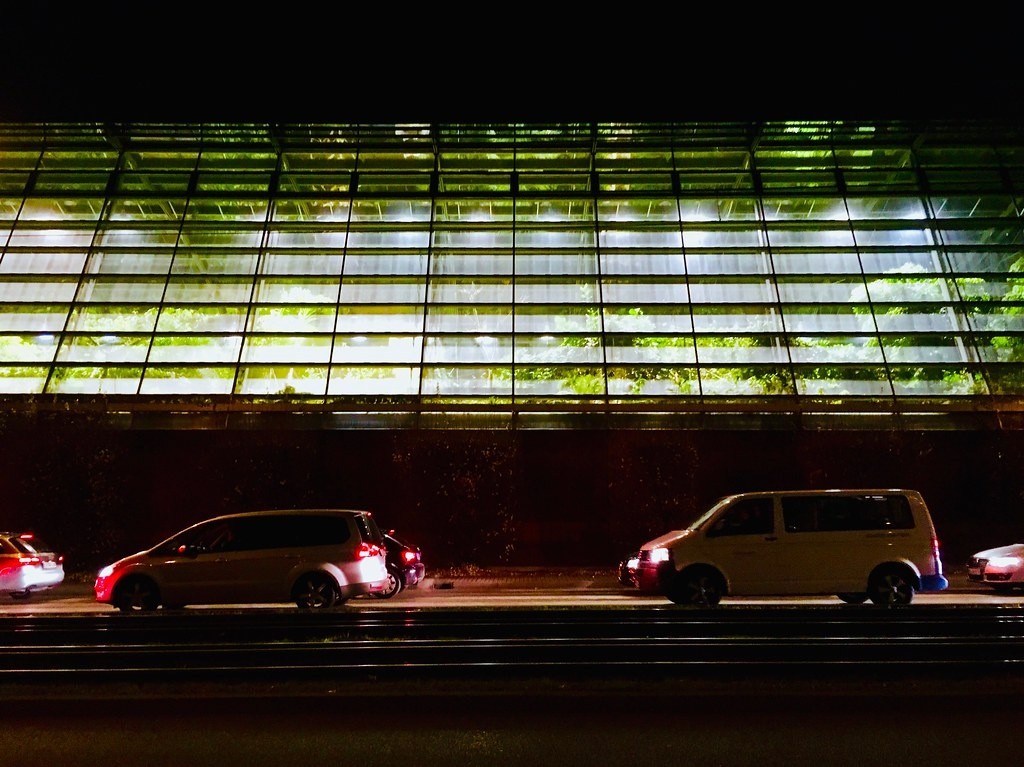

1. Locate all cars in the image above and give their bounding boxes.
[94,508,390,617]
[368,534,426,599]
[966,543,1024,596]
[0,529,66,600]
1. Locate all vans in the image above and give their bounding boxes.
[617,488,949,608]
